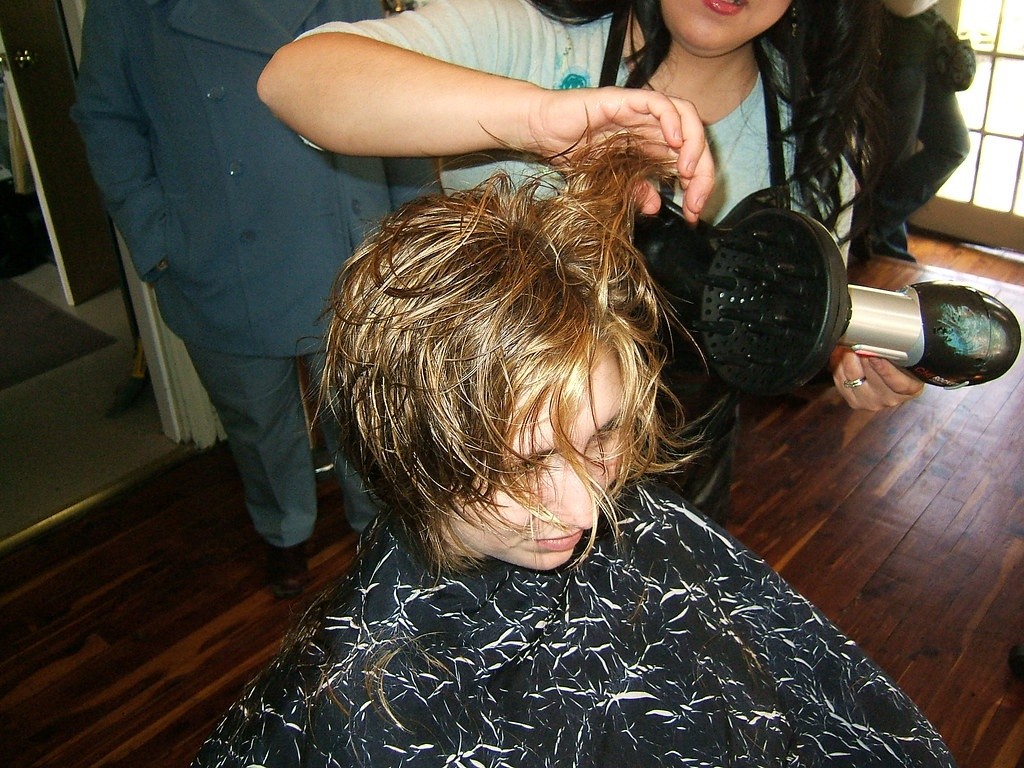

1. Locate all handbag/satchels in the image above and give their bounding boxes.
[932,37,975,91]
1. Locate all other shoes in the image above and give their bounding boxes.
[269,545,307,598]
[849,232,873,263]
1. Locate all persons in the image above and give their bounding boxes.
[189,188,958,768]
[256,0,925,532]
[849,0,971,268]
[68,0,442,600]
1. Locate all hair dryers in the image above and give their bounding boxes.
[692,209,1021,396]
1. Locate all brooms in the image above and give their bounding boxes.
[105,337,147,418]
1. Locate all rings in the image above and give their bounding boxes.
[843,377,866,388]
